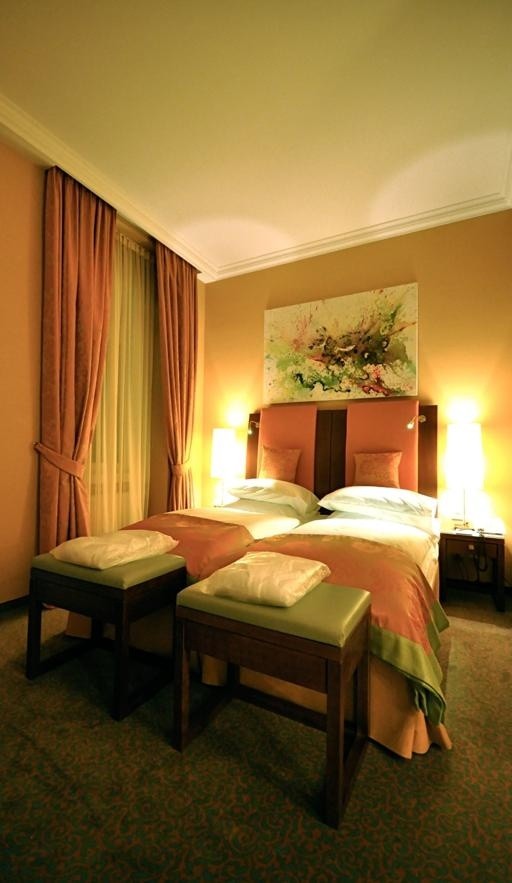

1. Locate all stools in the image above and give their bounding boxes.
[176,572,372,825]
[30,551,193,723]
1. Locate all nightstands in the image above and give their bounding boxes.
[440,530,504,612]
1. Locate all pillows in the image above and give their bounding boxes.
[48,529,179,568]
[226,445,439,522]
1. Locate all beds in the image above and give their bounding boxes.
[132,399,439,748]
